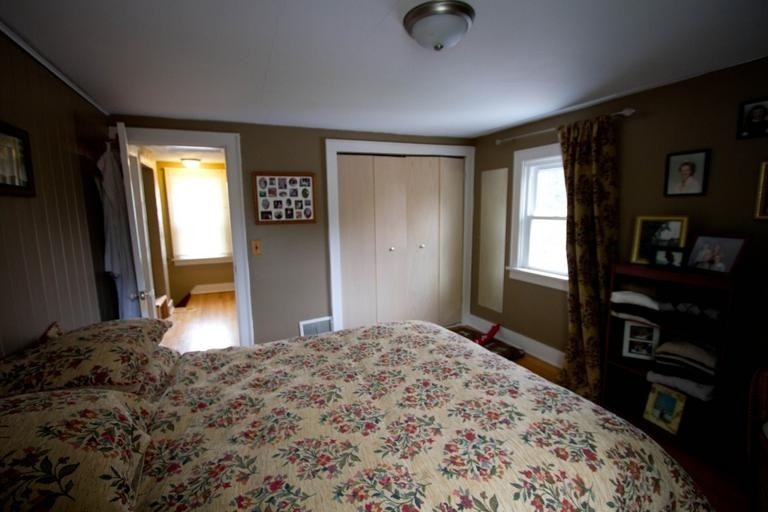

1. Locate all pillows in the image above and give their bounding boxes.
[0,320,181,511]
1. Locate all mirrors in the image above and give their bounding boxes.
[477,167,509,316]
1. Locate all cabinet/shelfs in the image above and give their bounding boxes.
[336,152,465,327]
[597,260,768,488]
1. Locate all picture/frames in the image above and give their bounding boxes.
[0,119,36,199]
[642,382,687,437]
[630,215,751,278]
[664,147,707,197]
[250,170,320,224]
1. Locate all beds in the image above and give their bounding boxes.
[0,320,712,512]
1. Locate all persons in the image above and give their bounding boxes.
[679,161,702,192]
[694,241,724,272]
